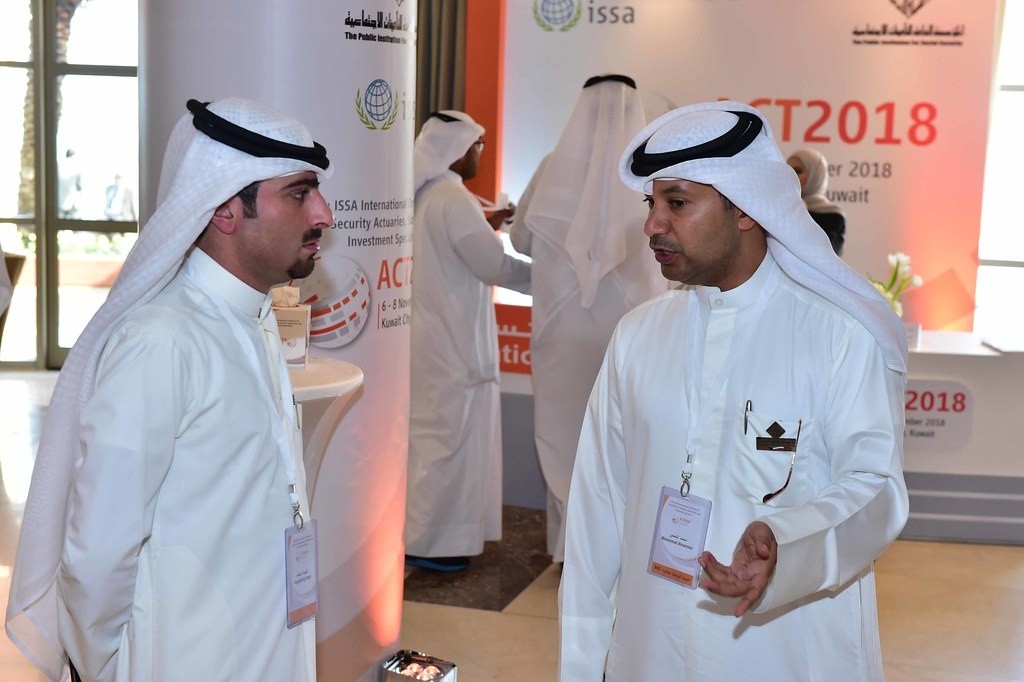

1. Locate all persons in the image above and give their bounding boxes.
[5,97,333,682]
[511,75,676,573]
[559,101,909,682]
[786,149,846,255]
[405,111,532,571]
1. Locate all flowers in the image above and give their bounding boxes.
[865,250,924,319]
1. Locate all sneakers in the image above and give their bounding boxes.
[403,554,468,571]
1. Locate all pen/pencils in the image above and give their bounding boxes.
[745,400,752,436]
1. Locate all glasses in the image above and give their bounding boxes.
[474,141,485,152]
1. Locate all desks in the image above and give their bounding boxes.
[895,330,1024,548]
[287,354,364,514]
